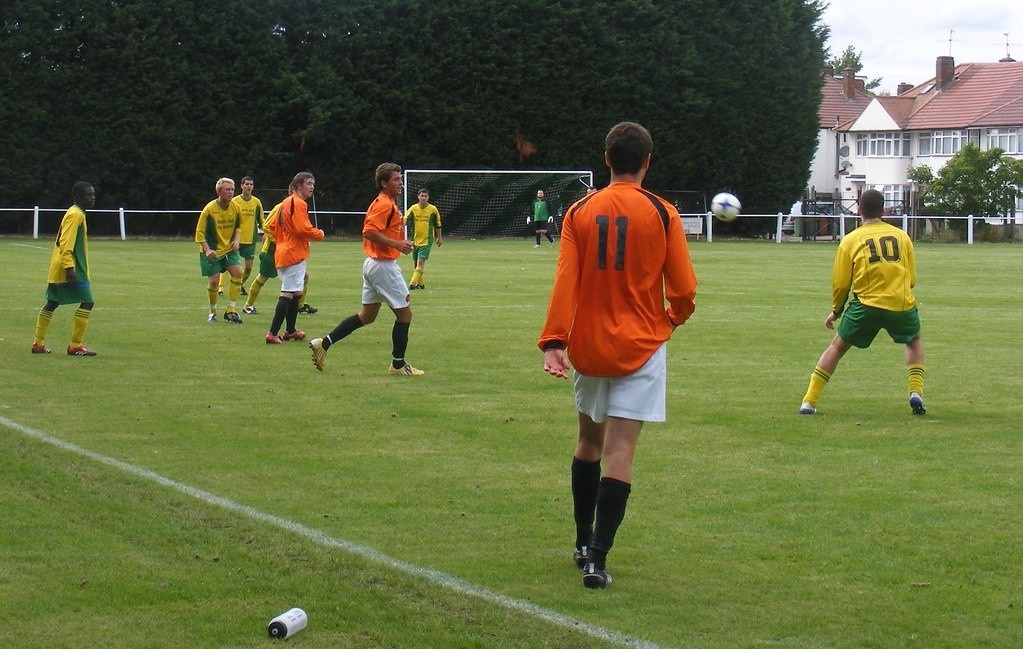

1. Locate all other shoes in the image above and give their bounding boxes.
[218,291,224,296]
[533,244,541,248]
[408,283,425,290]
[549,239,556,248]
[240,287,248,295]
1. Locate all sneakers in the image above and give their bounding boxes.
[282,329,306,340]
[207,314,219,325]
[223,311,243,324]
[242,306,258,314]
[67,345,97,356]
[908,392,927,416]
[298,303,318,314]
[31,342,52,354]
[265,332,285,344]
[572,547,592,568]
[799,403,817,414]
[309,338,329,371]
[387,362,425,376]
[582,563,613,590]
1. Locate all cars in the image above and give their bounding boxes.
[884,206,910,228]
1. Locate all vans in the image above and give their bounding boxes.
[782,201,855,235]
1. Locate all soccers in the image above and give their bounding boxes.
[710,193,742,223]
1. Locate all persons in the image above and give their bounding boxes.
[310,163,424,375]
[800,190,927,414]
[218,177,266,295]
[403,188,442,290]
[266,172,324,344]
[194,178,243,323]
[242,181,317,314]
[538,123,697,590]
[31,182,98,357]
[527,189,555,248]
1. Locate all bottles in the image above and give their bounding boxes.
[268,608,308,640]
